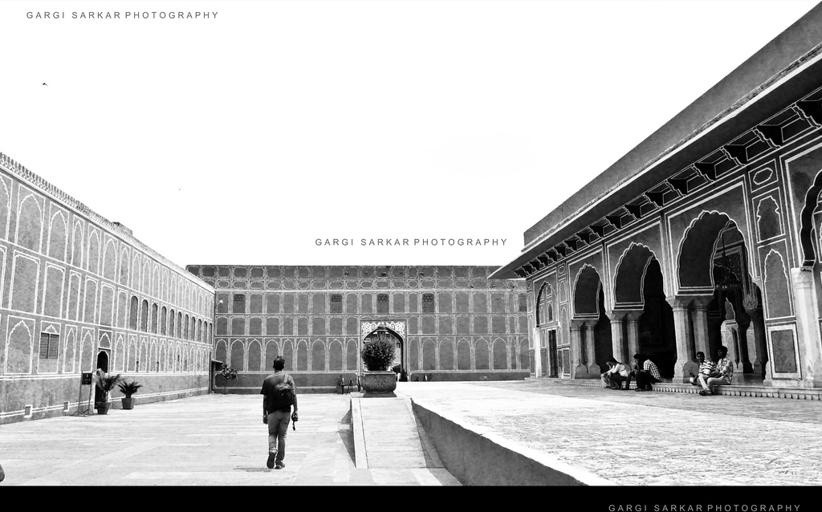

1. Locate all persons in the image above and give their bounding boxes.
[601,353,661,391]
[699,346,733,396]
[689,351,716,387]
[260,356,298,469]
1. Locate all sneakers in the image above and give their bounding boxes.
[275,462,285,469]
[267,453,275,468]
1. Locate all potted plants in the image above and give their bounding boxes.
[92,369,122,415]
[359,335,397,398]
[118,378,142,410]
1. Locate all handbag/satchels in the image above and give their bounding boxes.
[269,374,296,410]
